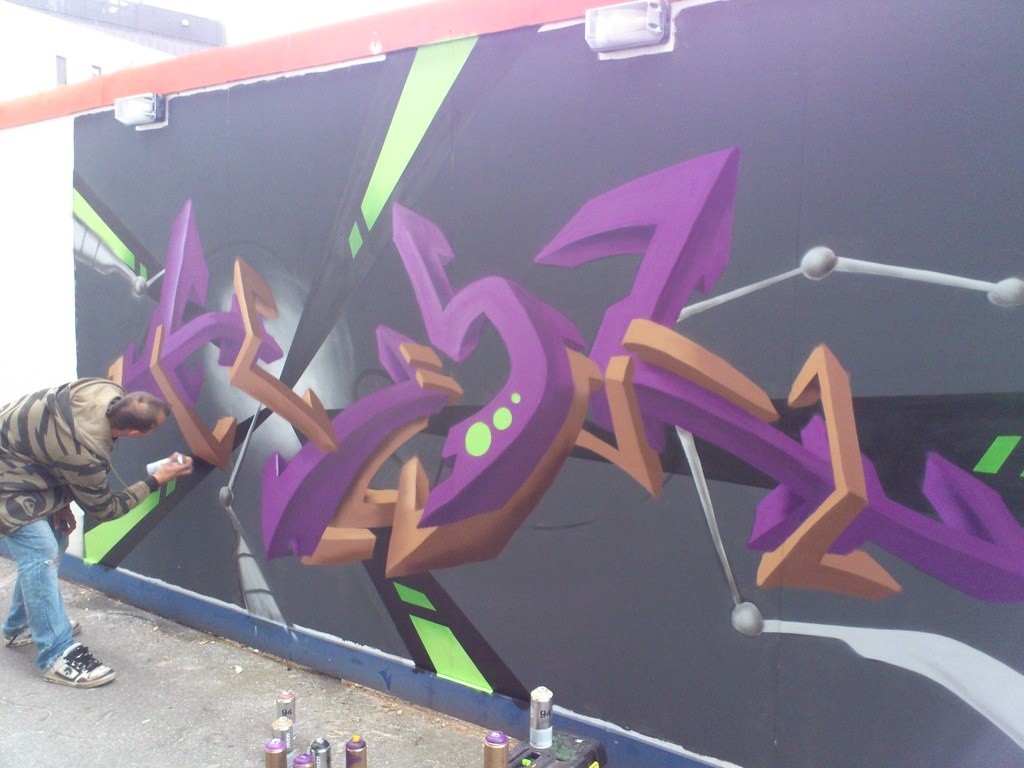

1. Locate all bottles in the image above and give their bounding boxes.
[265,737,288,768]
[482,731,508,768]
[530,686,552,749]
[276,689,296,739]
[147,454,190,477]
[273,716,295,753]
[311,737,332,768]
[293,753,314,768]
[346,734,367,768]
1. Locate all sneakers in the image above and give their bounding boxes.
[3,621,81,647]
[41,643,117,688]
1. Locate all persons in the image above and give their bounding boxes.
[0,376,193,689]
